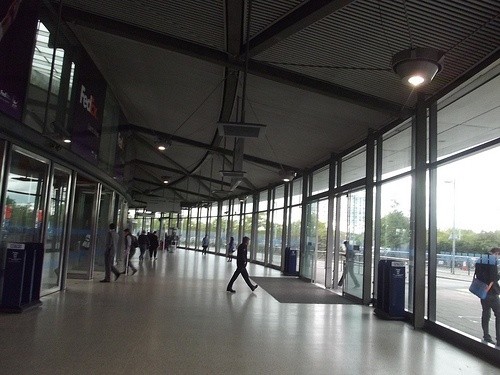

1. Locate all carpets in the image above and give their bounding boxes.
[249,275,357,305]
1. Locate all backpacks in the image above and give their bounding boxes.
[126,234,138,248]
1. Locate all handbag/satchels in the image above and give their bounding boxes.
[469,278,490,300]
[231,248,235,253]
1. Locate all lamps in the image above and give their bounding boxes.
[153,140,171,151]
[160,176,171,184]
[217,95,269,143]
[389,46,445,89]
[211,136,233,200]
[218,137,248,179]
[278,166,298,183]
[238,194,249,202]
[46,120,73,141]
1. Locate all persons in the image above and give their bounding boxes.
[475,247,500,347]
[338,241,361,288]
[97,224,179,282]
[226,237,234,262]
[202,234,208,255]
[226,236,258,293]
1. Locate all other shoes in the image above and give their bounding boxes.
[227,289,236,293]
[114,274,120,282]
[120,271,129,274]
[252,284,258,291]
[483,339,495,345]
[99,279,110,282]
[353,285,359,289]
[132,269,137,275]
